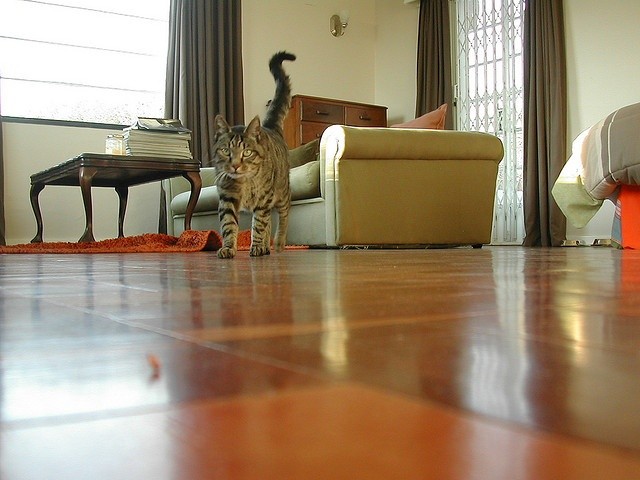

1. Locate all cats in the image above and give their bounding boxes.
[210,51,297,258]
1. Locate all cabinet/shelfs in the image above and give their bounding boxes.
[282,93,388,151]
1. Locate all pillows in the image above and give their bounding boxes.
[390,104,447,129]
[286,138,319,168]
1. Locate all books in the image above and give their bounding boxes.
[120,116,194,160]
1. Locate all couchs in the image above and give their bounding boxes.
[165,125,504,248]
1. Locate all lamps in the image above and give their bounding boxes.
[330,9,350,36]
